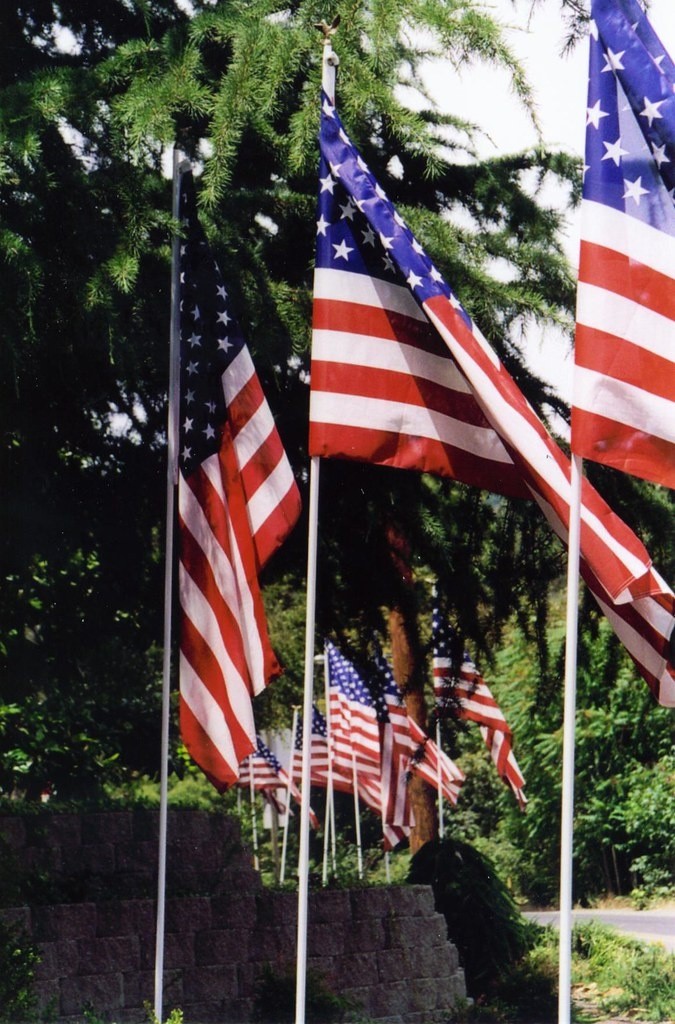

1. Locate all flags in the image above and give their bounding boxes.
[569,1,675,491]
[308,88,674,711]
[239,586,525,854]
[168,164,304,802]
